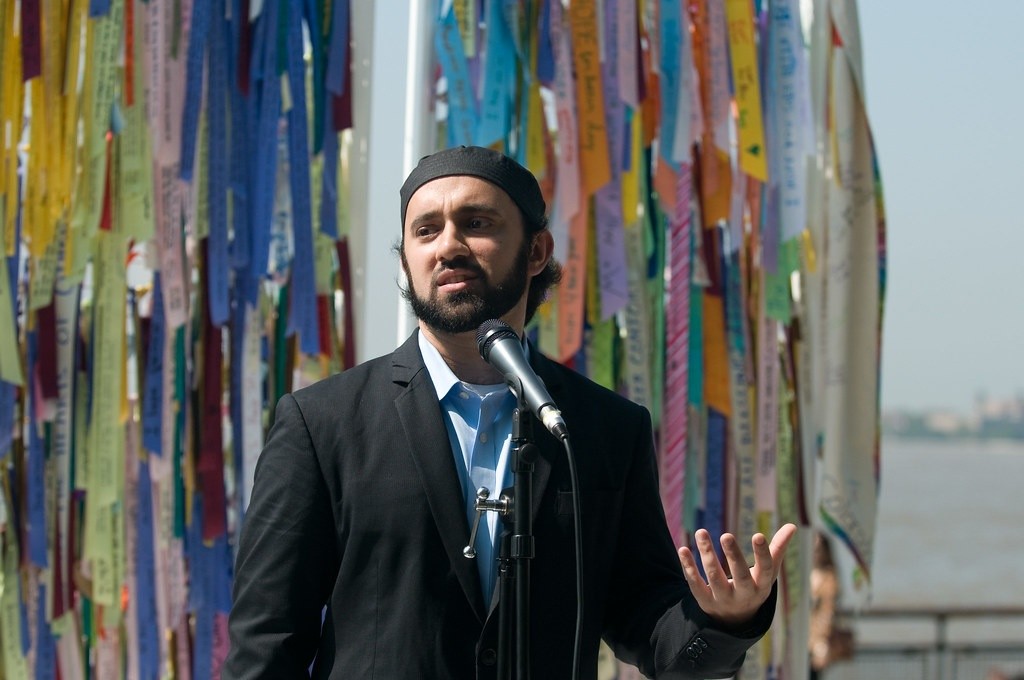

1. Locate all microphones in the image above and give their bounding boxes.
[475,319,569,443]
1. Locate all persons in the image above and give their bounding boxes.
[809,532,839,680]
[218,146,798,680]
